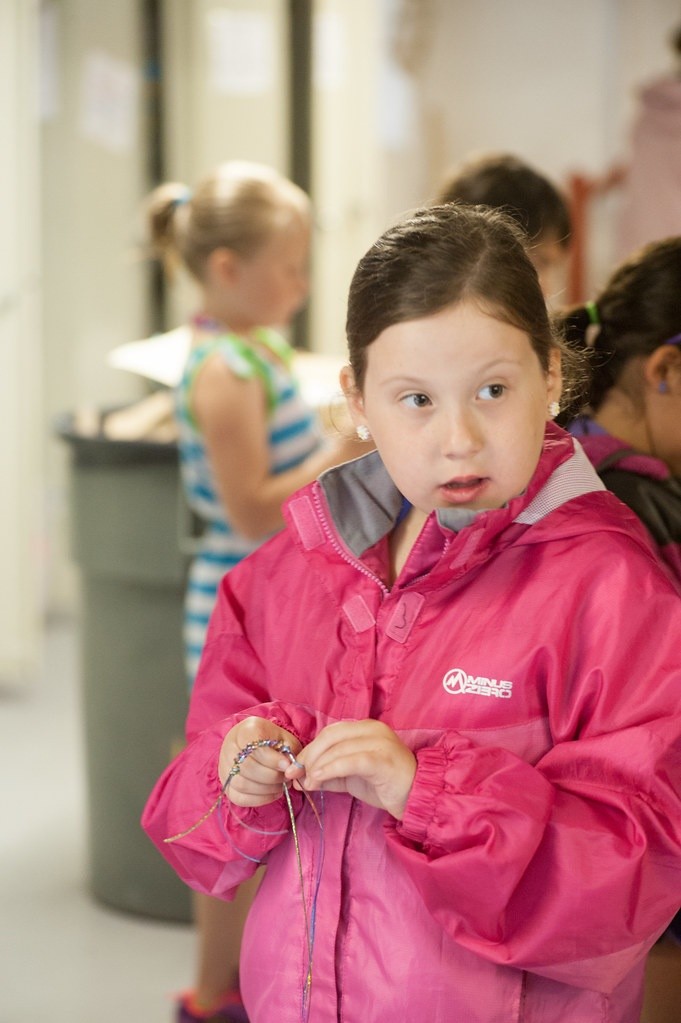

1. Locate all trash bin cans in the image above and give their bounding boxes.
[57,411,203,928]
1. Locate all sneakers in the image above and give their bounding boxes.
[176,989,250,1023]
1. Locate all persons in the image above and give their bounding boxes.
[441,161,574,277]
[173,168,364,1023]
[135,202,681,1022]
[535,239,681,581]
